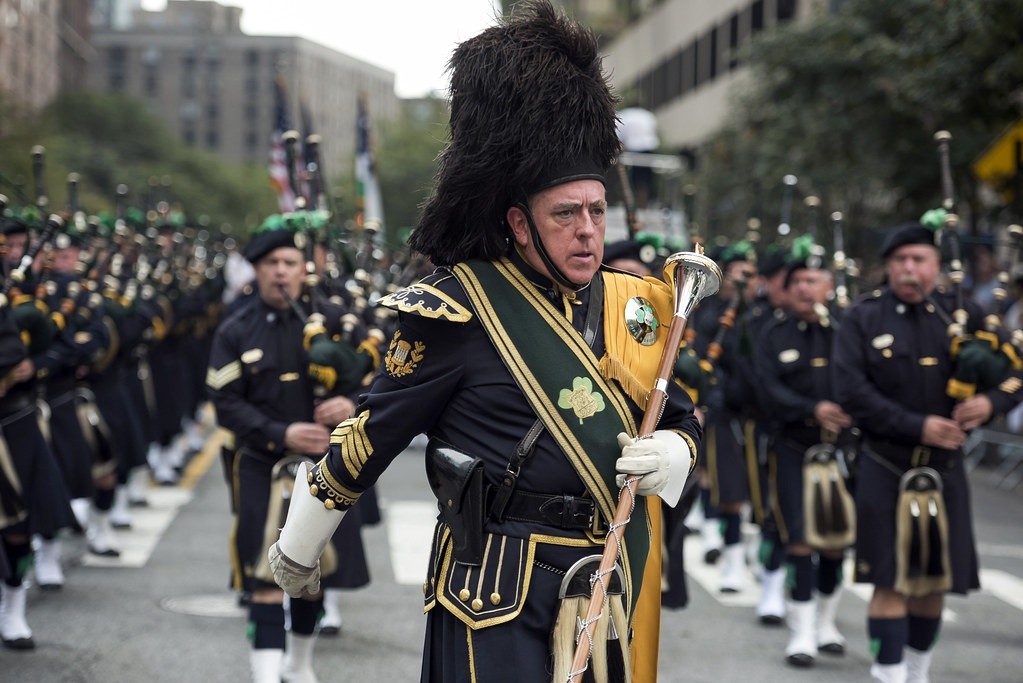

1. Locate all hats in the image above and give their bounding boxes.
[781,234,832,289]
[879,207,946,256]
[651,239,686,270]
[243,207,335,262]
[405,0,624,270]
[0,195,229,257]
[720,239,756,264]
[600,231,662,270]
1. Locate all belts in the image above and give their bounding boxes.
[485,486,608,535]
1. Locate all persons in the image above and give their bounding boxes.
[602,174,1023,683]
[269,1,704,683]
[0,192,437,683]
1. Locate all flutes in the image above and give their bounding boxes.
[569,251,724,683]
[0,129,1022,456]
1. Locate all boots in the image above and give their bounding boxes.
[318,589,342,633]
[282,592,292,631]
[756,565,786,622]
[906,647,934,683]
[870,662,908,683]
[813,589,847,651]
[682,493,707,534]
[784,596,817,663]
[744,522,761,564]
[718,543,747,592]
[282,634,319,683]
[250,648,282,683]
[1,419,207,650]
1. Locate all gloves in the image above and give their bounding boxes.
[268,542,322,600]
[616,430,670,496]
